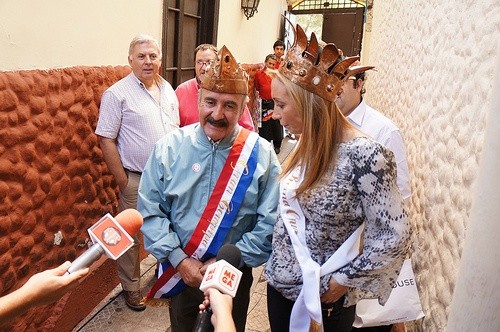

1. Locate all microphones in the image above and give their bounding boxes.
[68,206,144,274]
[193,244,244,332]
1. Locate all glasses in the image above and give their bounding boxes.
[195,60,212,65]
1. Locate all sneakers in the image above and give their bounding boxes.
[124,289,146,310]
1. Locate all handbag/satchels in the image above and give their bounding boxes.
[352,259,426,328]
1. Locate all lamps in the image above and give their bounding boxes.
[241,0,260,20]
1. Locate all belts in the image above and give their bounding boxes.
[130,169,142,175]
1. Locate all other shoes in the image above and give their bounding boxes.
[275,147,280,154]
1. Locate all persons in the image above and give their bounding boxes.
[136,45,282,332]
[266,14,413,332]
[200,287,236,332]
[176,44,254,131]
[0,262,90,320]
[334,59,410,332]
[273,40,285,70]
[255,54,283,153]
[95,35,181,311]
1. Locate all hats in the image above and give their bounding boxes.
[201,45,249,95]
[278,13,379,103]
